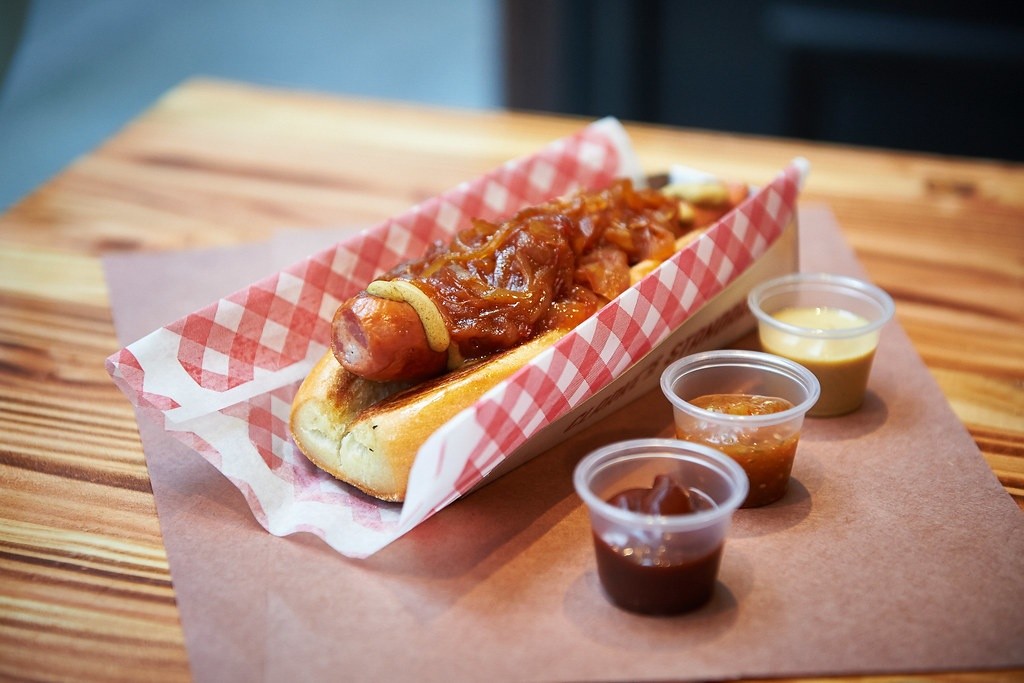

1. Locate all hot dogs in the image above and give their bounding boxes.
[287,177,752,505]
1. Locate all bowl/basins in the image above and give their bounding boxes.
[572,438,750,616]
[661,350,821,506]
[747,273,896,417]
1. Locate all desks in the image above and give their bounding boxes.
[0,75,1024,683]
